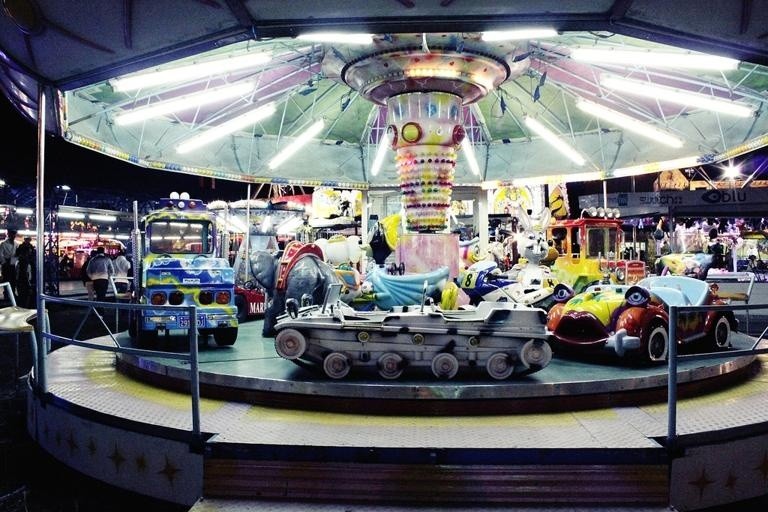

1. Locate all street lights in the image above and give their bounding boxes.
[49,184,72,247]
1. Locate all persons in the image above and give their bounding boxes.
[539,240,559,265]
[746,255,758,272]
[81,247,132,301]
[0,226,36,300]
